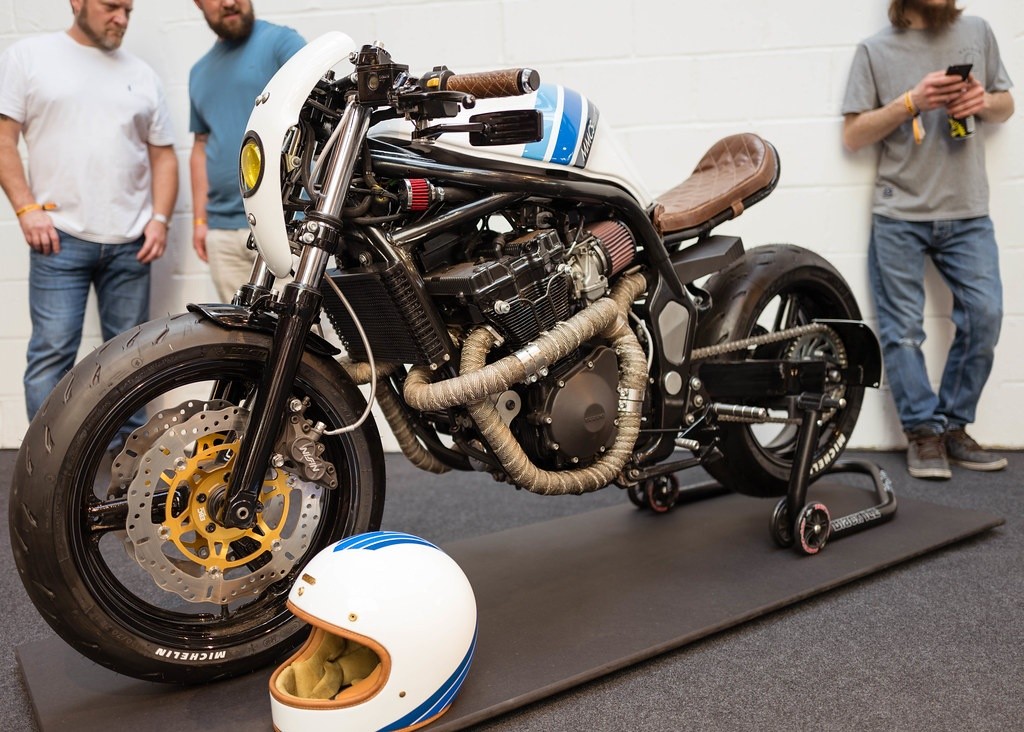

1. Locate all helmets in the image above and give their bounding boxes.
[268,530,478,732]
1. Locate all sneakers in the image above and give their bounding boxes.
[907,424,951,479]
[943,425,1008,471]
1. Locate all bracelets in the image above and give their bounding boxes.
[905,91,926,145]
[193,218,207,225]
[153,214,172,229]
[15,202,57,217]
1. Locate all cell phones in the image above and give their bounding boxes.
[945,63,973,81]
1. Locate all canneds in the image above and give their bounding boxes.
[947,87,976,141]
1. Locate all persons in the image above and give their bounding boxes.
[840,0,1015,478]
[188,0,308,305]
[0,0,178,459]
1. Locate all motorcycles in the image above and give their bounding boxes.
[7,31,899,688]
